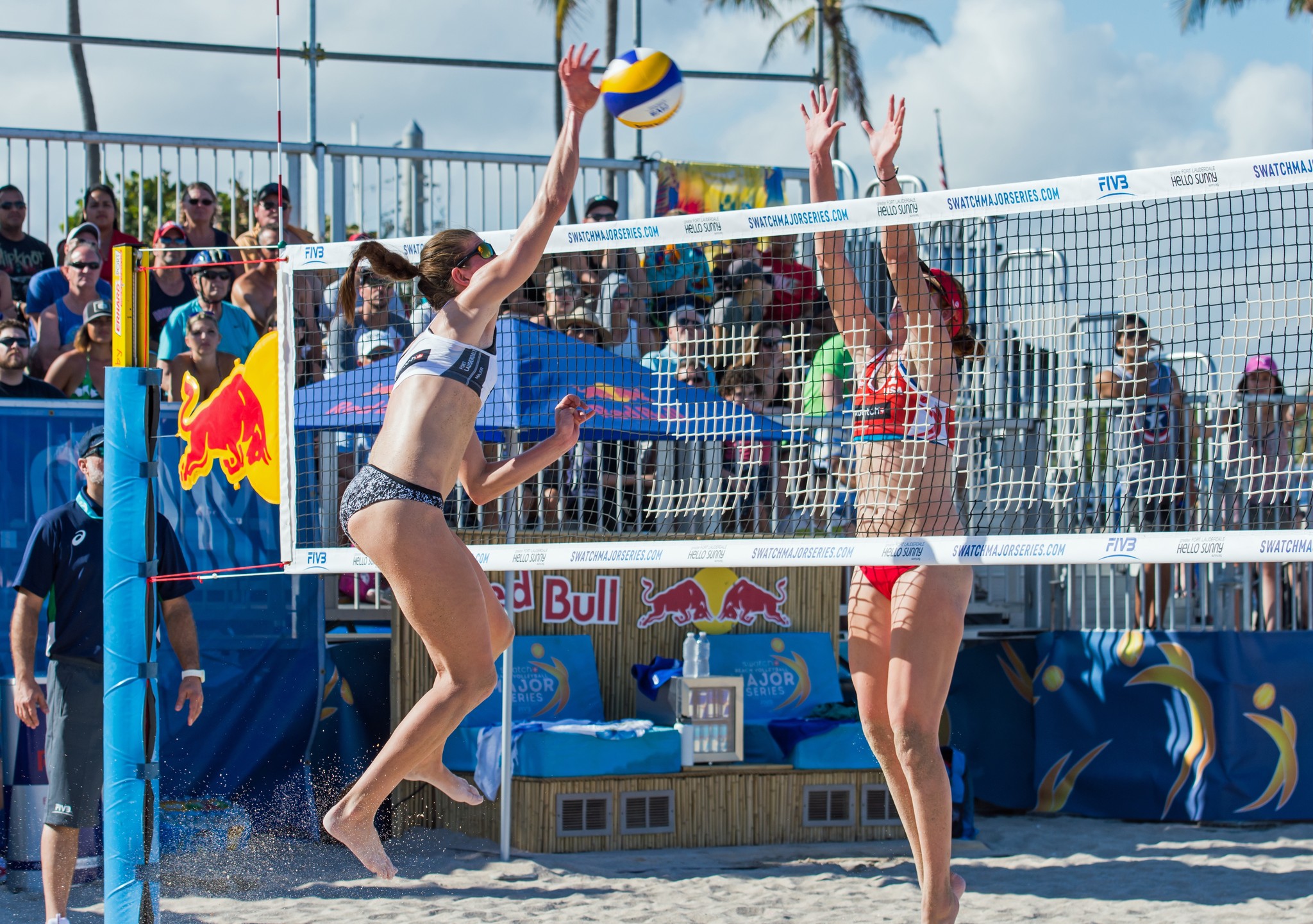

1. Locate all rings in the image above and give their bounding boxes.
[198,705,202,708]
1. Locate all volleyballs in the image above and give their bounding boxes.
[600,47,683,129]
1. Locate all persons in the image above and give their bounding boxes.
[800,84,985,924]
[0,183,1313,664]
[323,43,602,879]
[10,426,206,924]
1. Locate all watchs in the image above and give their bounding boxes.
[181,669,206,683]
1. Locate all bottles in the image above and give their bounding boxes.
[696,632,710,677]
[682,632,699,678]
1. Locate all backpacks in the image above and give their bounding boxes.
[939,744,980,841]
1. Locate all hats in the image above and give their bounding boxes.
[153,220,186,244]
[255,183,291,202]
[348,233,370,241]
[1114,314,1148,356]
[886,256,963,339]
[553,306,611,346]
[584,195,618,216]
[357,329,394,356]
[82,299,112,325]
[725,259,762,282]
[65,221,102,249]
[545,266,577,287]
[78,424,104,456]
[1244,356,1279,378]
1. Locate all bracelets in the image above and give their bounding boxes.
[873,165,899,187]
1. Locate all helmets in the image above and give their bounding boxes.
[191,249,233,273]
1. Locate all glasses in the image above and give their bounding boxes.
[187,197,214,205]
[586,212,615,221]
[570,325,598,337]
[438,242,496,289]
[735,391,755,397]
[554,289,578,299]
[68,261,100,270]
[258,201,291,211]
[366,278,394,288]
[80,445,104,458]
[366,348,394,359]
[734,237,758,246]
[188,311,215,318]
[1,201,26,210]
[70,239,97,246]
[613,292,635,301]
[680,377,704,384]
[158,237,186,246]
[1124,330,1148,340]
[762,338,786,349]
[678,318,703,330]
[201,271,231,280]
[751,275,764,282]
[272,319,306,328]
[0,337,30,348]
[265,244,277,250]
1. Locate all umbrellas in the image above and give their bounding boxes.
[296,318,817,861]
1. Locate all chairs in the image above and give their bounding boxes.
[442,636,968,855]
